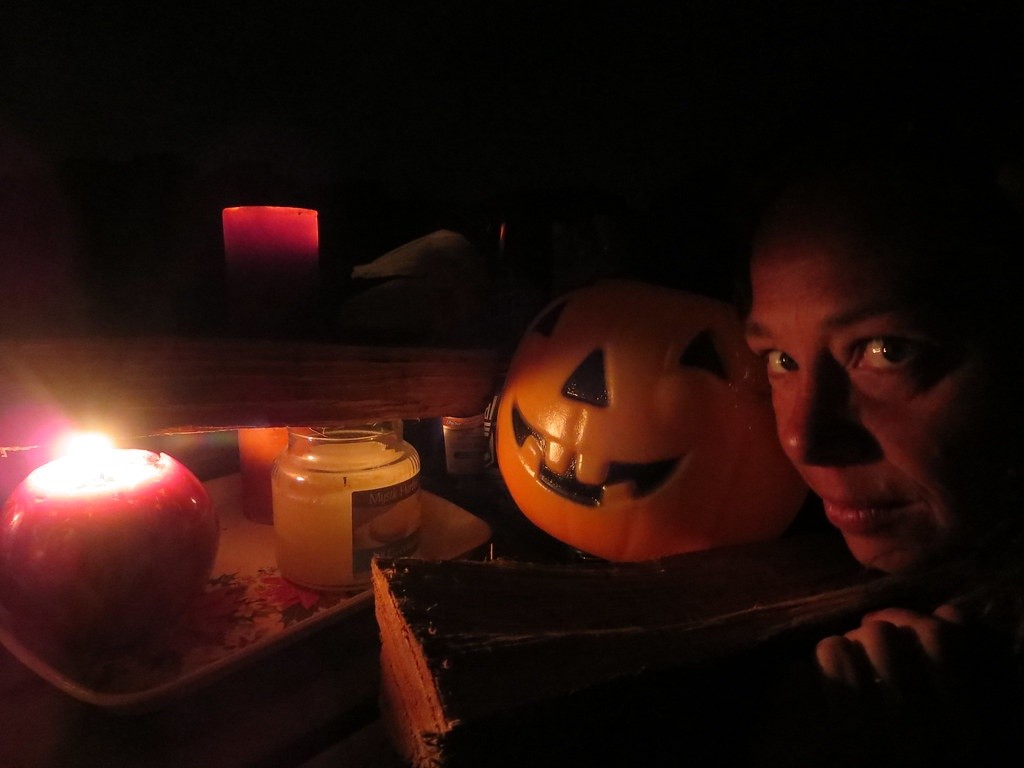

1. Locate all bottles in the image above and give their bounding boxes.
[273,418,422,594]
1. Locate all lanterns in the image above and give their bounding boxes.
[492,280,812,559]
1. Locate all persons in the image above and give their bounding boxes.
[746,167,1024,768]
